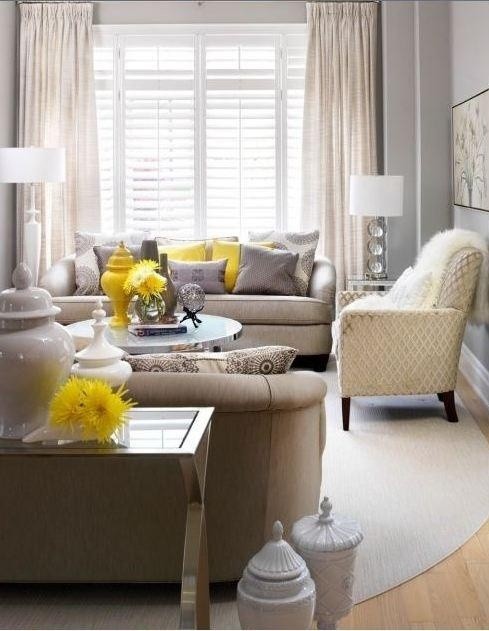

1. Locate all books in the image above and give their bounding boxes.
[128,324,186,336]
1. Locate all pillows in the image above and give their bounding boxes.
[122,345,299,374]
[74,229,319,296]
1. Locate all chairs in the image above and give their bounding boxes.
[336,228,486,431]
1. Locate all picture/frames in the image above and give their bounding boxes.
[452,88,489,212]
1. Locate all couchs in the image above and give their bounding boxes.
[0,369,326,587]
[39,253,336,372]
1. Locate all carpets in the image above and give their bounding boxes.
[1,325,489,630]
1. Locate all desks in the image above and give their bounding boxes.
[64,312,243,364]
[0,405,215,630]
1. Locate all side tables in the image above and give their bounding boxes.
[347,274,397,291]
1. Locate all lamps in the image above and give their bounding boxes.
[0,145,67,290]
[349,175,404,279]
